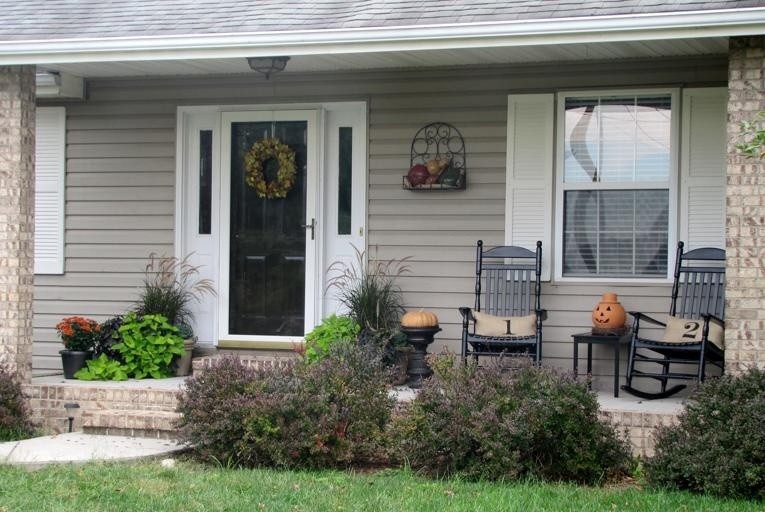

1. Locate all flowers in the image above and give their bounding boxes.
[52,316,103,352]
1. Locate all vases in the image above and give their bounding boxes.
[59,348,91,379]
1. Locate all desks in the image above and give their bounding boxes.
[569,327,636,398]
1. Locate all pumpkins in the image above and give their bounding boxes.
[408,159,459,187]
[593,302,626,328]
[401,308,438,328]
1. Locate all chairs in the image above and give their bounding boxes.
[617,239,726,402]
[458,238,549,374]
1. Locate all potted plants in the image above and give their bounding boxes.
[166,321,199,376]
